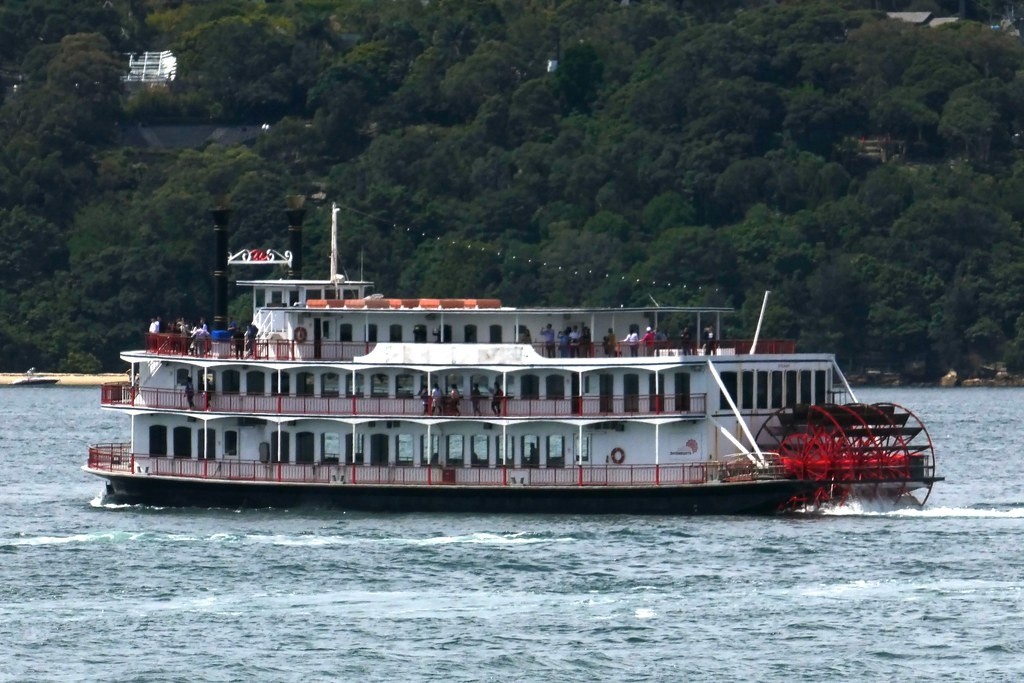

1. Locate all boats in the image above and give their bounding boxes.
[79,201,937,519]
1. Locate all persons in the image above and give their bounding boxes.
[430,383,444,416]
[418,385,432,415]
[528,443,537,463]
[680,327,693,354]
[521,329,533,344]
[489,382,503,416]
[469,383,483,416]
[556,317,670,359]
[447,383,462,416]
[143,313,261,359]
[125,369,140,383]
[702,324,715,355]
[202,378,212,409]
[540,323,555,357]
[183,377,195,411]
